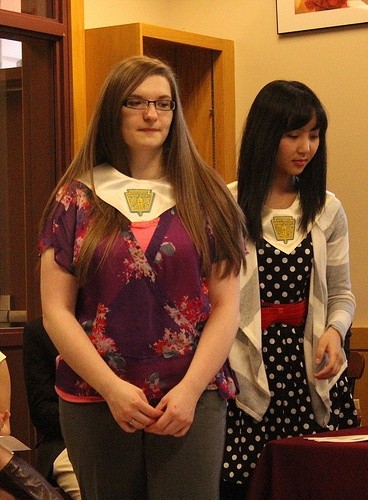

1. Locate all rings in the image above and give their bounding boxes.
[130,419,134,425]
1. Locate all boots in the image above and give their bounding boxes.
[0,454,69,500]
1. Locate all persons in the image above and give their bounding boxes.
[221,80,360,484]
[0,316,81,500]
[39,55,248,500]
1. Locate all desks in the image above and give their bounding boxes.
[250,425,368,500]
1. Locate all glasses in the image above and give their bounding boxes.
[122,99,176,111]
